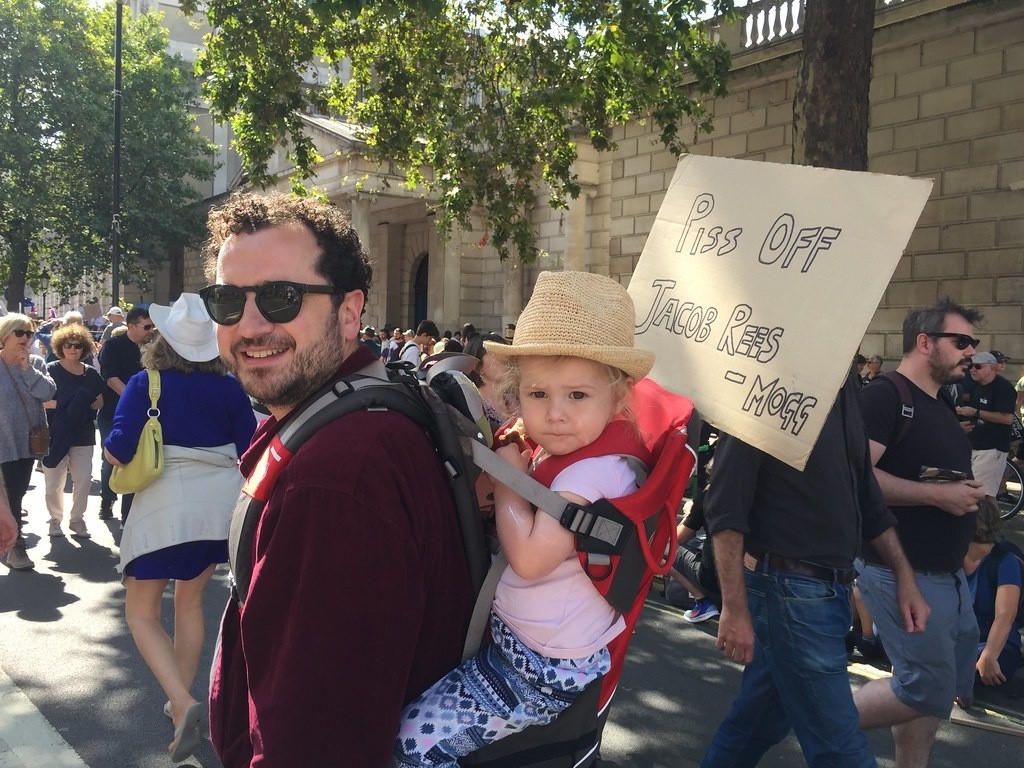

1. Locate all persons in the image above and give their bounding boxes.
[856,353,883,389]
[0,312,58,570]
[845,575,883,659]
[659,451,720,623]
[28,307,125,471]
[1014,375,1024,514]
[851,300,983,767]
[990,350,1017,506]
[98,306,154,530]
[204,193,479,768]
[398,320,439,381]
[702,335,931,768]
[947,352,1018,511]
[47,323,103,538]
[359,322,515,381]
[0,467,19,560]
[963,501,1023,706]
[393,269,655,767]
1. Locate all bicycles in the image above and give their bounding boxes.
[995,458,1024,522]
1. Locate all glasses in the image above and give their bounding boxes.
[63,343,83,349]
[12,330,34,338]
[867,361,876,363]
[968,363,993,369]
[925,332,980,350]
[138,323,156,330]
[997,359,1008,364]
[199,281,350,326]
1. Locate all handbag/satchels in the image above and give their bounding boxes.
[29,423,51,455]
[108,369,163,494]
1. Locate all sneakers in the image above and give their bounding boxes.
[6,537,35,571]
[995,489,1019,506]
[684,598,720,623]
[118,514,128,529]
[99,499,115,520]
[69,520,91,538]
[47,518,63,537]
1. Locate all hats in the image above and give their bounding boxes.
[482,270,655,384]
[402,330,415,337]
[989,350,1012,360]
[105,306,123,316]
[971,352,997,364]
[360,326,378,336]
[148,292,220,362]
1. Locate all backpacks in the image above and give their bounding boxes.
[985,537,1024,630]
[389,344,419,362]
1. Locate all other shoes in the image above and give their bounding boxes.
[956,695,972,708]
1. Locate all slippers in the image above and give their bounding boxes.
[163,699,203,762]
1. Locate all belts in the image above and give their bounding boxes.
[746,543,860,584]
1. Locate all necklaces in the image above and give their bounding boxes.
[100,291,257,763]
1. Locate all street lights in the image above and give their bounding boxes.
[38,266,50,322]
